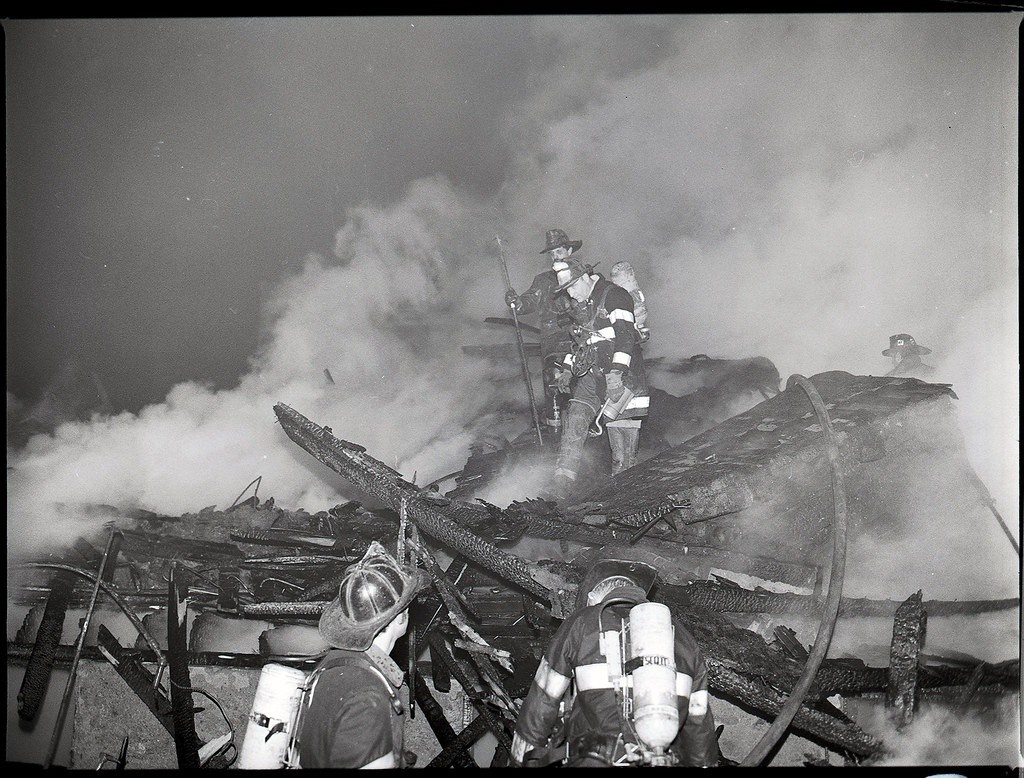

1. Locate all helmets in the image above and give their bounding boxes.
[575,558,658,613]
[318,555,432,651]
[539,229,582,255]
[552,259,602,296]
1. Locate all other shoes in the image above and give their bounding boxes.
[535,476,573,502]
[549,424,562,442]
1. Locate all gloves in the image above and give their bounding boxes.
[511,730,536,763]
[604,371,625,405]
[505,287,521,310]
[558,369,574,394]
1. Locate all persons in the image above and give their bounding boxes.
[508,559,719,767]
[882,334,933,383]
[544,258,650,502]
[285,555,432,768]
[505,228,594,453]
